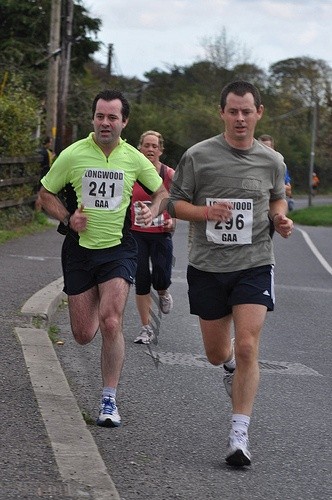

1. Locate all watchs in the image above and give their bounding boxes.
[63,213,72,229]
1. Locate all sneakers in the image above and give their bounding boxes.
[159,289,173,314]
[225,429,251,467]
[134,326,158,345]
[221,339,236,396]
[96,394,121,428]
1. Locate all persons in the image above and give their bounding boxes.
[40,136,56,180]
[130,130,177,344]
[40,90,170,427]
[168,80,294,464]
[259,135,320,214]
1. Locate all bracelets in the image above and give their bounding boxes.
[271,213,279,223]
[205,206,210,222]
